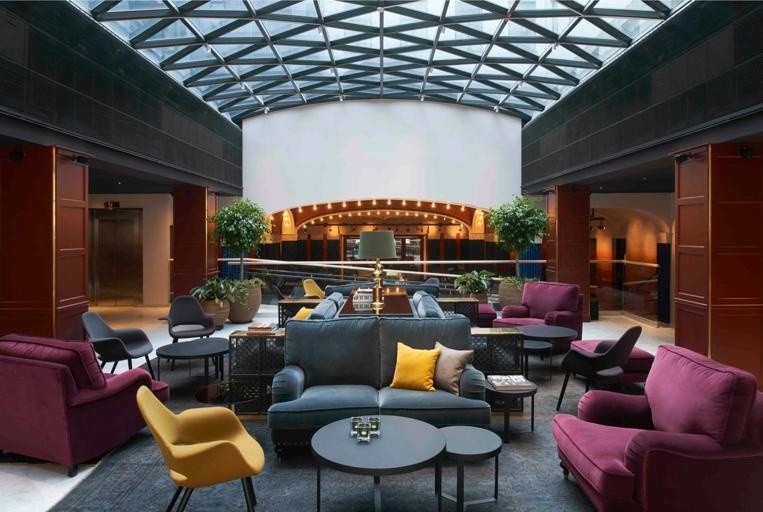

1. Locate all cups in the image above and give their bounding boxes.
[350,416,380,443]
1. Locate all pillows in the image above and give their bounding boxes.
[391,342,442,393]
[431,342,475,396]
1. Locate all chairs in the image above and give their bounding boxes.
[156,296,218,343]
[557,326,642,410]
[82,311,156,381]
[137,385,266,512]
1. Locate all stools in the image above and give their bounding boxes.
[571,338,656,392]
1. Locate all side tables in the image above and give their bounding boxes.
[484,379,539,434]
[435,425,502,508]
[518,340,556,381]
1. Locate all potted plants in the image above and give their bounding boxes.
[480,182,555,315]
[198,192,279,324]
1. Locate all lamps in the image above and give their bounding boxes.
[354,226,400,316]
[587,205,607,232]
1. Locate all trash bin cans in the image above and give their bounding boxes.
[589,285,602,320]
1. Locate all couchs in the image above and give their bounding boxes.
[492,280,581,330]
[277,273,493,320]
[553,345,763,512]
[0,333,169,476]
[267,317,493,463]
